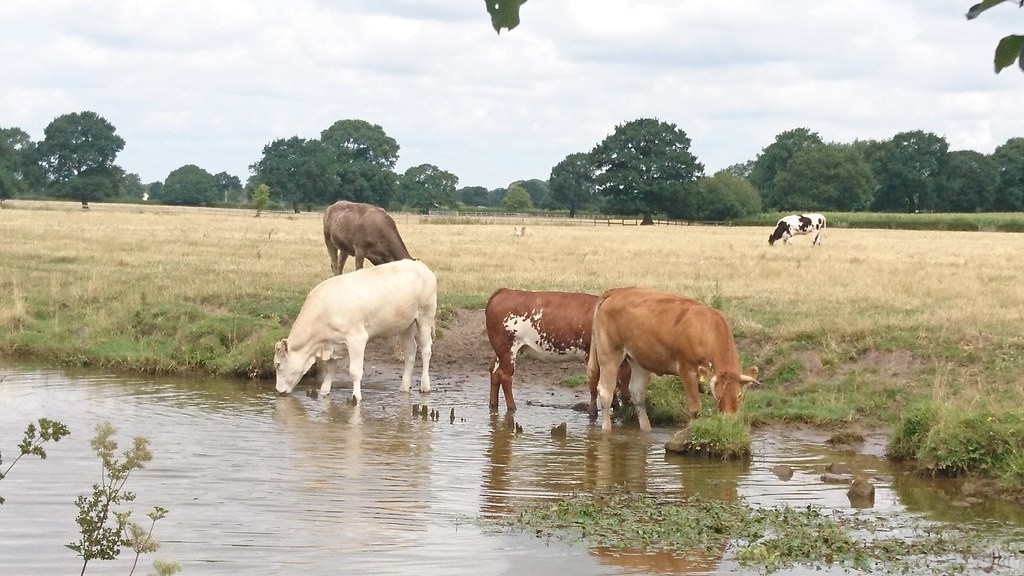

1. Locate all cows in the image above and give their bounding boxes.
[514,226,533,238]
[767,213,827,247]
[323,200,414,277]
[485,286,633,415]
[271,259,439,403]
[586,285,764,432]
